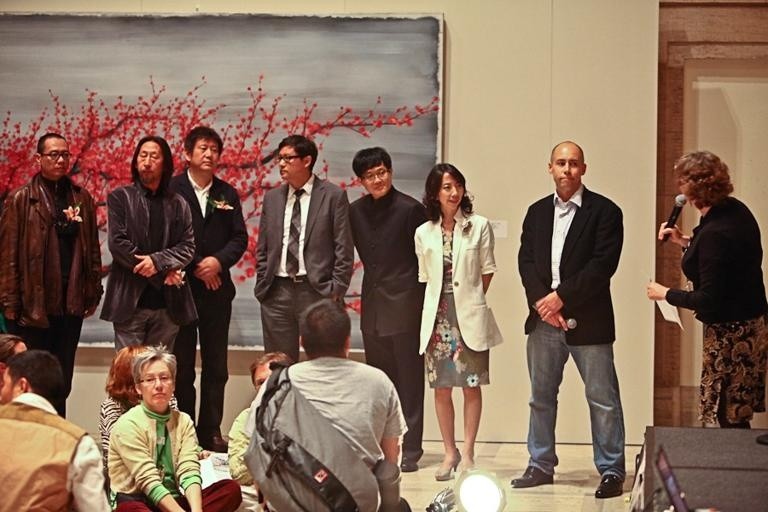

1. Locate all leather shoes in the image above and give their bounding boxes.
[195,428,228,453]
[511,466,553,487]
[595,474,622,498]
[401,443,418,472]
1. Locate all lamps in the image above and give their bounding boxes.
[426,466,507,512]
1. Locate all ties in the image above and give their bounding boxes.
[286,189,306,280]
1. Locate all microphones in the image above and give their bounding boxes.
[565,318,577,329]
[663,193,688,242]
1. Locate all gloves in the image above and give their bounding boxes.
[426,487,456,512]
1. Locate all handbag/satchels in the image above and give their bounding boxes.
[242,360,382,512]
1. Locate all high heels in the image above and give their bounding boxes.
[435,448,461,481]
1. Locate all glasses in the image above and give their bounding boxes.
[275,154,300,162]
[358,169,390,181]
[42,150,72,161]
[137,374,169,384]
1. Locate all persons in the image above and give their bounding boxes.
[647,150,768,430]
[2,126,503,481]
[510,141,627,497]
[3,297,411,511]
